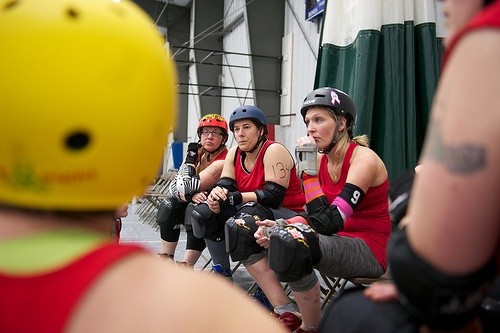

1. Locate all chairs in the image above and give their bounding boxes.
[135,168,408,311]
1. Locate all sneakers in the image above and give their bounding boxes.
[292,327,319,333]
[271,311,302,332]
[211,263,233,281]
[175,260,194,270]
[249,286,272,310]
[157,252,174,261]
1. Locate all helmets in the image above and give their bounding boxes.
[301,87,356,122]
[197,114,228,139]
[0,0,180,210]
[229,105,268,134]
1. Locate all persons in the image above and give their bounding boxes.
[0,0,290,333]
[224,87,393,333]
[191,104,307,311]
[312,0,500,333]
[156,113,229,270]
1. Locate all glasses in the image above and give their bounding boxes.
[201,130,225,137]
